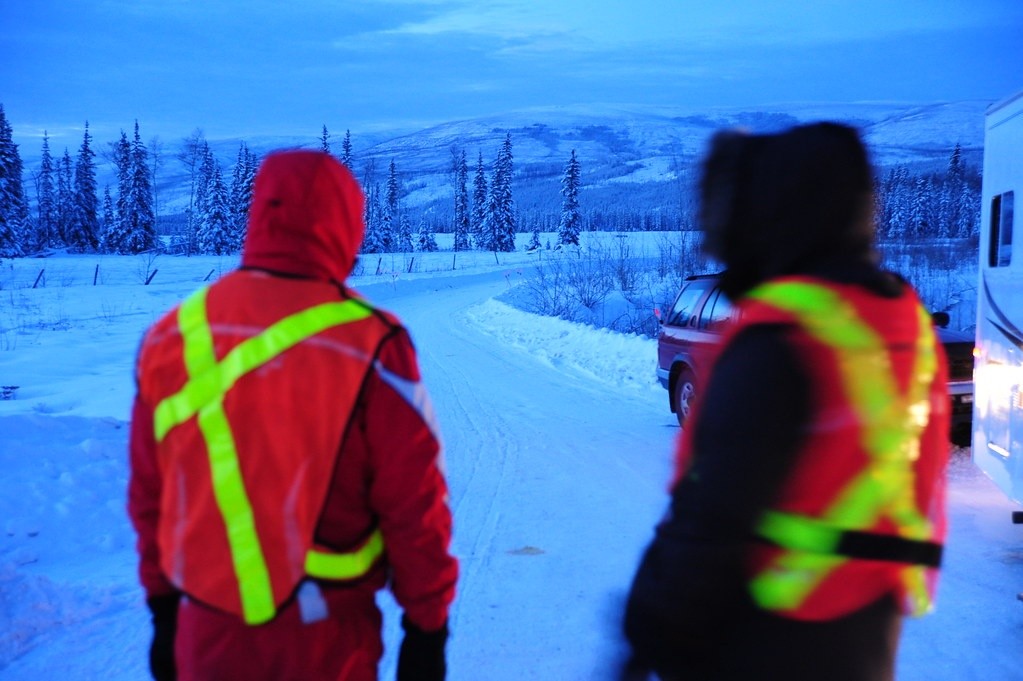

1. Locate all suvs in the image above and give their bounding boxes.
[654,273,975,450]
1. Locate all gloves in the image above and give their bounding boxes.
[396,614,449,681]
[147,592,176,681]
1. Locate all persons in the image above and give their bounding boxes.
[593,122,951,681]
[127,149,460,681]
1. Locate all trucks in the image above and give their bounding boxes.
[969,88,1023,534]
